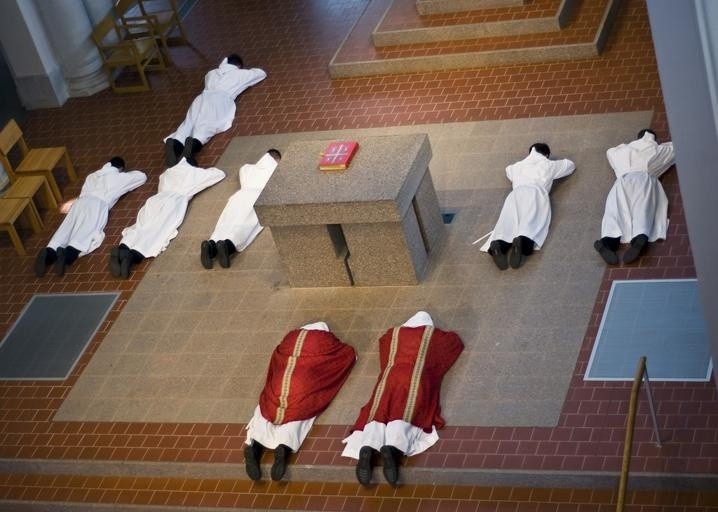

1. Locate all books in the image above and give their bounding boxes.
[319,141,359,170]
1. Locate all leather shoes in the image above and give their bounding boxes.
[379,449,399,486]
[489,241,509,271]
[508,236,524,270]
[34,246,50,278]
[243,444,264,483]
[107,244,123,280]
[269,444,289,483]
[54,246,67,278]
[214,239,232,269]
[198,237,215,270]
[163,138,179,166]
[592,240,619,266]
[117,246,134,281]
[182,134,199,166]
[353,446,373,488]
[621,234,651,266]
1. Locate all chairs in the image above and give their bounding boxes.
[91,10,166,93]
[112,0,183,64]
[0,117,77,202]
[0,199,43,256]
[0,149,58,234]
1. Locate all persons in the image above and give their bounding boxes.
[244,321,358,482]
[110,156,226,278]
[36,157,147,277]
[471,143,575,270]
[163,53,266,168]
[594,129,675,265]
[200,148,281,269]
[341,311,464,485]
